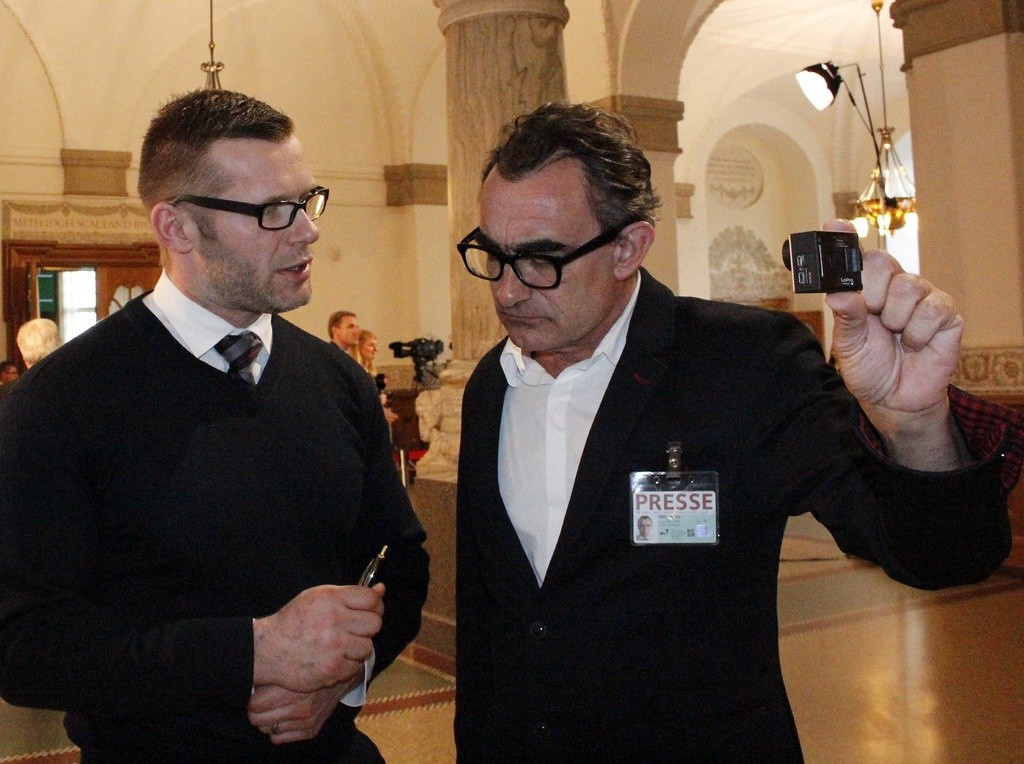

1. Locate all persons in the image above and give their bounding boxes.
[635,515,654,541]
[0,89,427,764]
[451,100,1024,764]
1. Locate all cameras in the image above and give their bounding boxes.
[782,230,863,293]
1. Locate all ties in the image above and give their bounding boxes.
[214,330,263,385]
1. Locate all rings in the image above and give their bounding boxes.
[271,723,282,736]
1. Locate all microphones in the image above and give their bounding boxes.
[388,341,416,349]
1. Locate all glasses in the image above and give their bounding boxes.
[172,186,329,231]
[458,212,655,290]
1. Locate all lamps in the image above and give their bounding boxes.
[850,0,918,240]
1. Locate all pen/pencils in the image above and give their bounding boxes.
[358,544,390,589]
